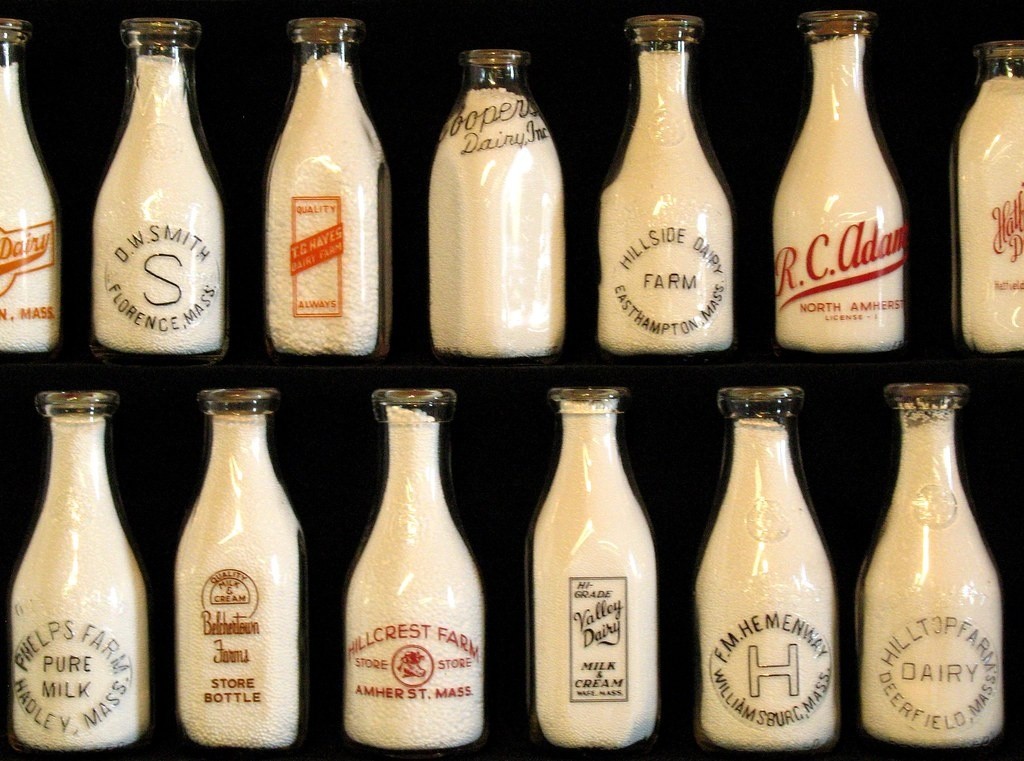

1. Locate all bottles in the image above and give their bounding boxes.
[695,387,846,753]
[88,16,222,355]
[170,385,312,751]
[268,16,392,351]
[8,391,155,749]
[853,376,1005,747]
[591,14,731,351]
[0,19,59,349]
[526,391,664,751]
[771,10,907,347]
[951,41,1024,360]
[431,45,563,357]
[339,391,484,749]
